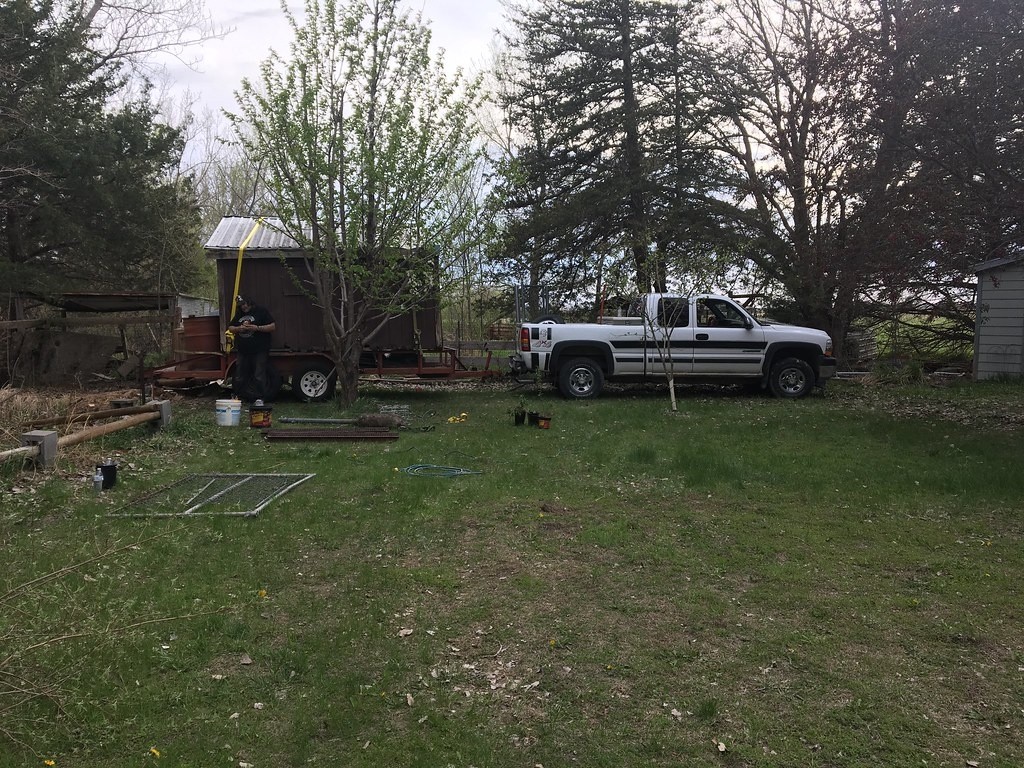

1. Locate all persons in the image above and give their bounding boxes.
[229,295,275,405]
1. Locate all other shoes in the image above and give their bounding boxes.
[261,394,273,402]
[253,399,264,406]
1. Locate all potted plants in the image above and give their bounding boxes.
[527,409,540,426]
[507,394,527,426]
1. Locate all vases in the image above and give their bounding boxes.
[96,462,117,488]
[538,417,551,429]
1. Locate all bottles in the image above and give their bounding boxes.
[93,468,104,492]
[105,457,113,465]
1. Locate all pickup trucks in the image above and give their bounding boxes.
[508,293,837,400]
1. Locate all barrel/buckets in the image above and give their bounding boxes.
[248,405,273,428]
[95,464,118,489]
[215,399,241,426]
[182,314,222,372]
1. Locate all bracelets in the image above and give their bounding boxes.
[257,326,263,332]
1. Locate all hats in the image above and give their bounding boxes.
[235,293,251,307]
